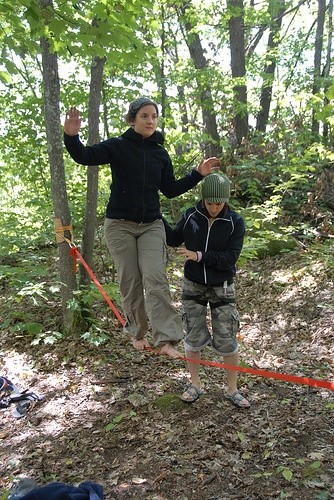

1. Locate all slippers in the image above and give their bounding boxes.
[0,375,44,420]
[223,390,252,408]
[180,385,204,402]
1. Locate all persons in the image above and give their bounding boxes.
[64,98,220,359]
[161,173,251,407]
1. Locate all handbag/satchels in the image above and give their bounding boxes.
[8,476,104,500]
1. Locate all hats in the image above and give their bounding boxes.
[201,174,232,204]
